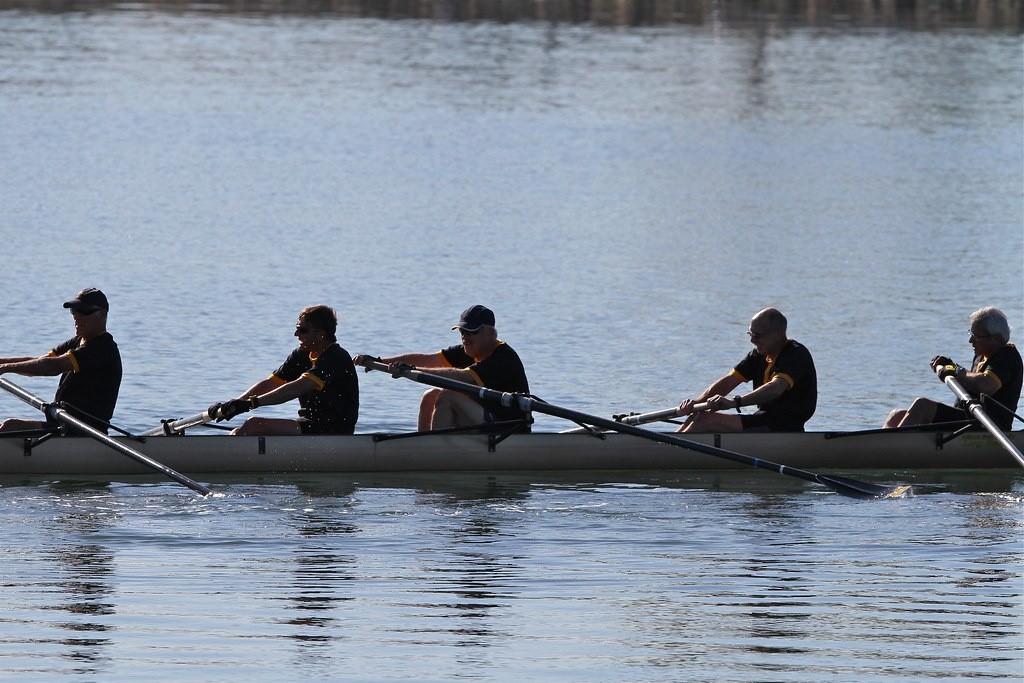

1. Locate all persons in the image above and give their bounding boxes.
[676,308,817,433]
[352,305,530,432]
[881,307,1024,431]
[208,305,359,436]
[0,288,122,435]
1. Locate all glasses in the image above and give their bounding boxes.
[296,323,320,334]
[459,328,480,335]
[70,307,102,316]
[967,330,994,341]
[746,331,778,339]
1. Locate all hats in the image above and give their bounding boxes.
[452,305,495,331]
[63,288,109,311]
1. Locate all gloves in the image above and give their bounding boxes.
[938,365,956,383]
[208,401,226,423]
[930,355,954,373]
[221,399,252,421]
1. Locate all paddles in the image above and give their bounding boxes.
[935,363,1024,469]
[561,401,718,434]
[137,405,236,436]
[358,356,884,500]
[1,376,218,496]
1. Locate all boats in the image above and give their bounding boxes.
[0,412,1024,478]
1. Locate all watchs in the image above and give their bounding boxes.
[733,395,742,413]
[955,366,964,376]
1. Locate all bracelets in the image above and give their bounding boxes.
[249,395,259,409]
